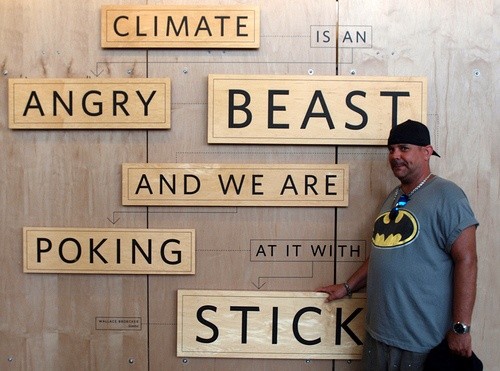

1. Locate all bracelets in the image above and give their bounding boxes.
[342,279,355,299]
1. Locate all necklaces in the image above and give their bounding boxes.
[389,172,432,205]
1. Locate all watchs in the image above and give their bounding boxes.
[448,321,471,335]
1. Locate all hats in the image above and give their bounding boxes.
[388,119,441,158]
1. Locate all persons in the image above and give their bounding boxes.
[316,118,484,371]
